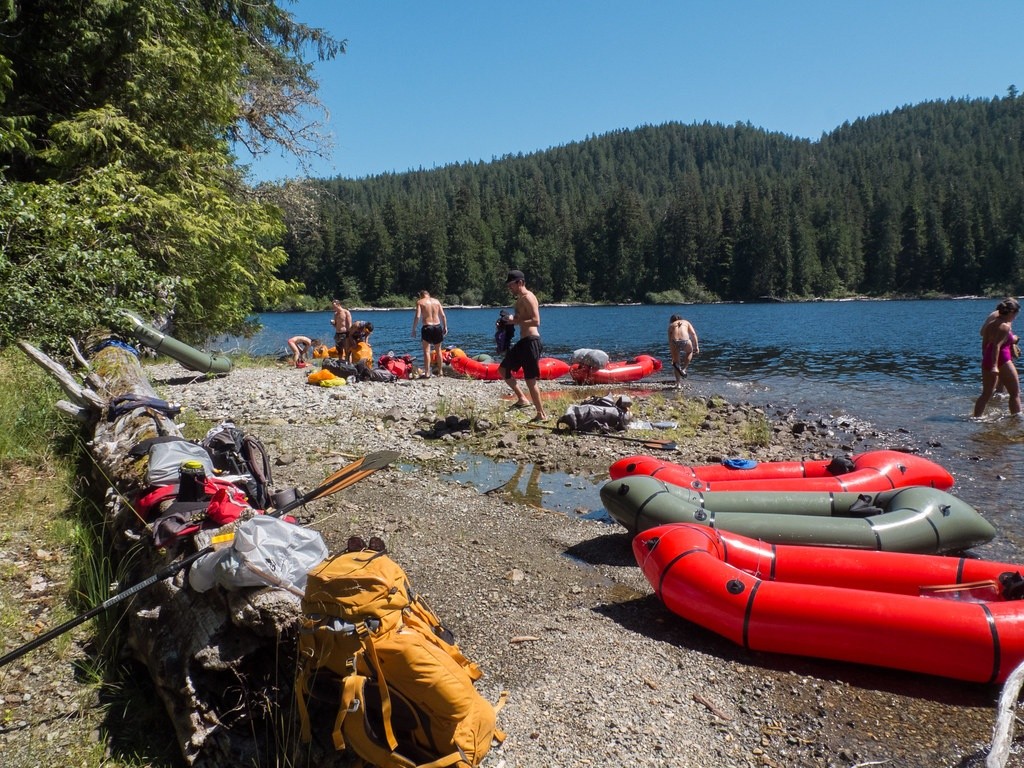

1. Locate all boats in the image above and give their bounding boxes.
[632,522,1024,691]
[450,352,569,383]
[600,475,997,558]
[569,348,661,382]
[431,345,494,367]
[610,448,956,493]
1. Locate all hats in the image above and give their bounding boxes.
[505,270,525,282]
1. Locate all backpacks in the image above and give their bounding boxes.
[207,428,273,515]
[558,392,633,434]
[294,548,511,768]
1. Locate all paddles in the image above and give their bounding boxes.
[1,446,402,669]
[524,423,679,450]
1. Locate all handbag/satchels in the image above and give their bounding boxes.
[213,514,329,596]
[196,423,235,447]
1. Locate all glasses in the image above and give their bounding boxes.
[331,536,387,561]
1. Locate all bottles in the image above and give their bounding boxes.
[175,461,207,503]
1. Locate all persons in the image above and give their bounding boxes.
[288,336,321,363]
[498,271,547,420]
[974,297,1023,417]
[411,291,447,378]
[668,315,699,388]
[495,310,511,353]
[331,300,375,365]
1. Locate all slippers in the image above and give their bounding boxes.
[529,418,549,423]
[508,402,530,411]
